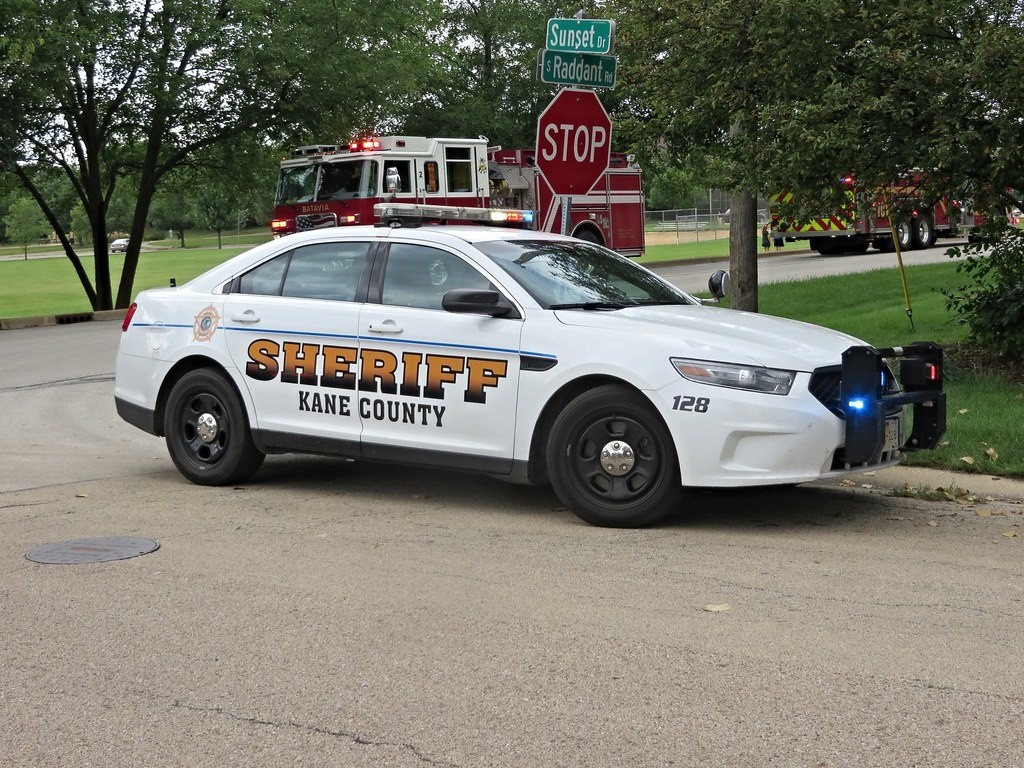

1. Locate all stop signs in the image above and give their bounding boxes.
[532,88,613,197]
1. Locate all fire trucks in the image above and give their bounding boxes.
[269,133,648,265]
[759,161,1024,256]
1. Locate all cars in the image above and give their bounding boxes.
[111,200,947,531]
[110,238,130,253]
